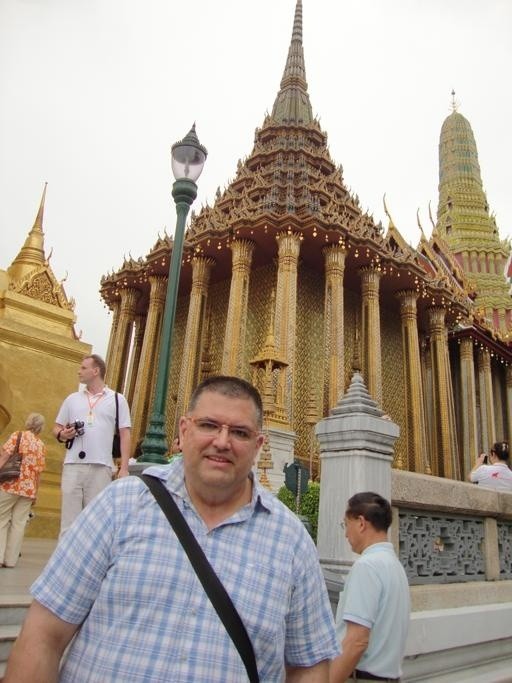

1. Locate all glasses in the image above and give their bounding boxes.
[340,517,357,529]
[186,416,259,444]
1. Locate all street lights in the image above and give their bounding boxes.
[136,124,207,463]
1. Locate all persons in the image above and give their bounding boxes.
[0,377,342,683]
[0,413,47,568]
[167,436,182,462]
[128,437,145,464]
[52,354,131,542]
[467,442,512,492]
[335,492,412,683]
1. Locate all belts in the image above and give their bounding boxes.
[348,670,390,680]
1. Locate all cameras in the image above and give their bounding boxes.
[68,420,86,437]
[484,456,488,463]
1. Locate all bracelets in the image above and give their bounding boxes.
[57,430,64,443]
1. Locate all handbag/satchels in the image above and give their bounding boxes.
[0,453,21,482]
